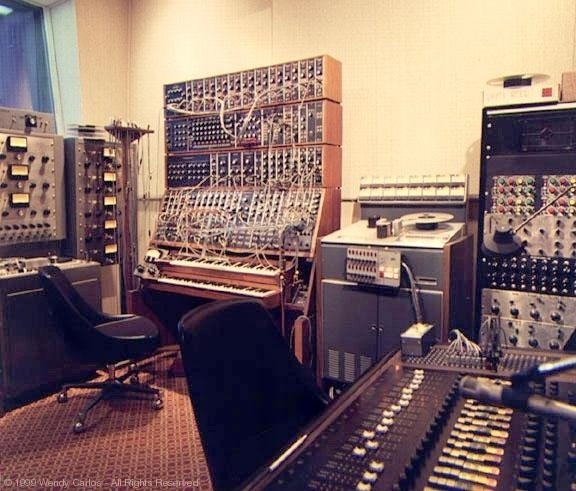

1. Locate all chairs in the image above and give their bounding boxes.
[176,294,354,490]
[38,264,164,434]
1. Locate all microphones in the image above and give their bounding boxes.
[458,373,576,420]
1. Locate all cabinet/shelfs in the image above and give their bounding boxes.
[314,174,481,393]
[1,257,103,414]
